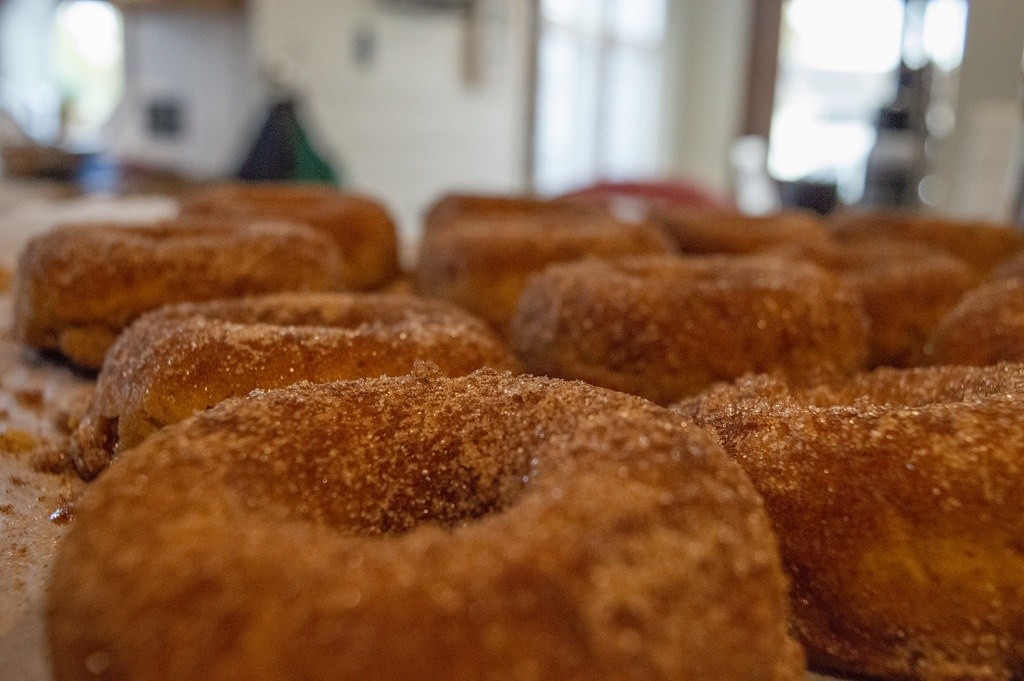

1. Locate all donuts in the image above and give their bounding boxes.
[16,179,1024,681]
